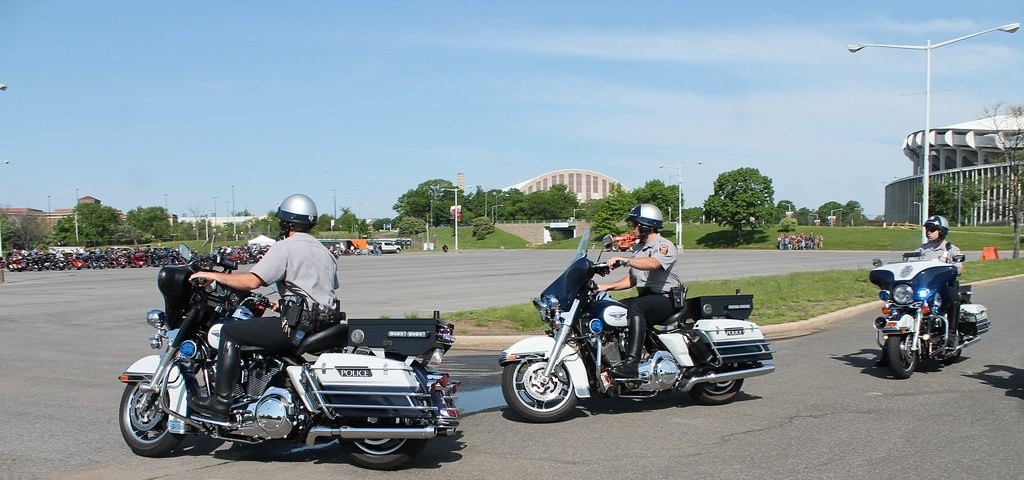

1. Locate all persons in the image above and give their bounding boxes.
[373,243,377,255]
[591,204,689,378]
[908,215,963,347]
[366,243,368,255]
[378,245,382,255]
[185,194,345,422]
[777,232,823,250]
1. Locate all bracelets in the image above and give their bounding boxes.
[627,257,630,266]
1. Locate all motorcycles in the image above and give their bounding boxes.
[499,225,775,423]
[118,243,462,472]
[0,243,271,272]
[600,232,642,252]
[869,251,992,379]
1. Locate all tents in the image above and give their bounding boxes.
[248,235,276,244]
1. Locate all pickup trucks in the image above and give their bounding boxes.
[367,243,402,253]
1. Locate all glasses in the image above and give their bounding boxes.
[633,220,642,228]
[927,228,941,233]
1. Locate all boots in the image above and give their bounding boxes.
[611,317,647,377]
[947,304,959,346]
[186,341,242,422]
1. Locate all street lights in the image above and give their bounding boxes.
[830,209,846,227]
[164,194,168,214]
[492,204,503,225]
[441,185,471,254]
[809,214,818,226]
[225,201,230,224]
[659,162,702,254]
[846,22,1021,245]
[212,196,219,224]
[47,195,51,220]
[331,189,336,220]
[913,202,921,226]
[783,203,790,217]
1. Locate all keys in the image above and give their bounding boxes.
[281,319,290,338]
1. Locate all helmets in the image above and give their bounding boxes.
[274,194,318,224]
[625,203,663,229]
[923,215,949,234]
